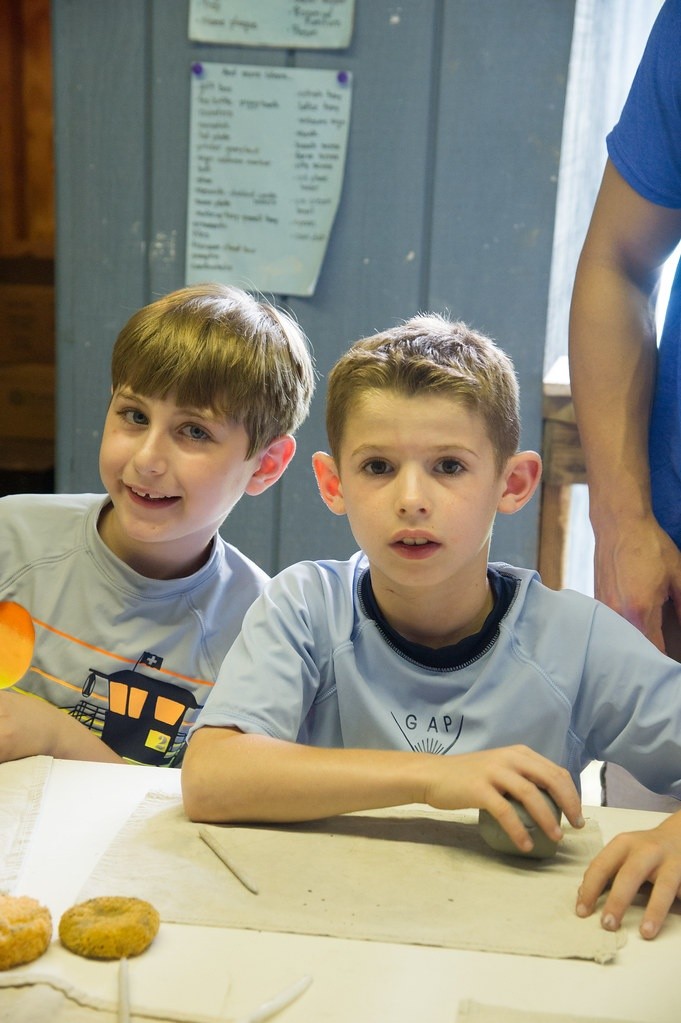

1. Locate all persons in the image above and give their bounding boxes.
[0,287,316,766]
[567,1,681,812]
[180,315,681,941]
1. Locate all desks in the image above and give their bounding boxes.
[0,751,681,1023]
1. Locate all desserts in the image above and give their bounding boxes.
[0,891,160,972]
